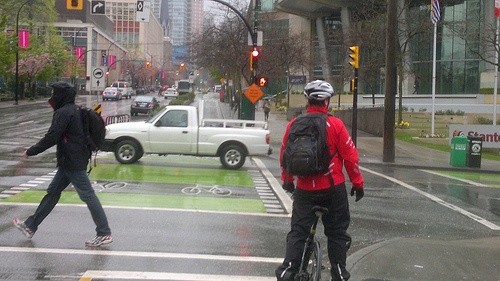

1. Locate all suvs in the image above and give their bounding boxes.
[110,81,133,99]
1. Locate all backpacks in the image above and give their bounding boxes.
[78,106,107,173]
[283,111,338,193]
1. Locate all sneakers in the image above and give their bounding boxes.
[84,234,113,247]
[13,218,35,239]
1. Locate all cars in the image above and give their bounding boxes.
[130,97,160,116]
[135,79,194,99]
[102,86,122,101]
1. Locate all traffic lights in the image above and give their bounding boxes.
[145,62,151,70]
[179,63,185,72]
[258,77,268,88]
[348,45,359,68]
[252,47,259,61]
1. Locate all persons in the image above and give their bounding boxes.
[13,82,112,248]
[263,99,270,120]
[276,80,364,281]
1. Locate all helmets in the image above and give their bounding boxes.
[303,79,334,102]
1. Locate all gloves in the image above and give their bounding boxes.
[351,187,364,202]
[282,182,294,194]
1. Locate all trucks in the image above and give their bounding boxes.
[215,85,221,93]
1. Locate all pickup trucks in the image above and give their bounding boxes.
[99,104,273,170]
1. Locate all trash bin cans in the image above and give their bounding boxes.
[450,135,483,168]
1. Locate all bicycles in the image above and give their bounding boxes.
[283,186,329,281]
